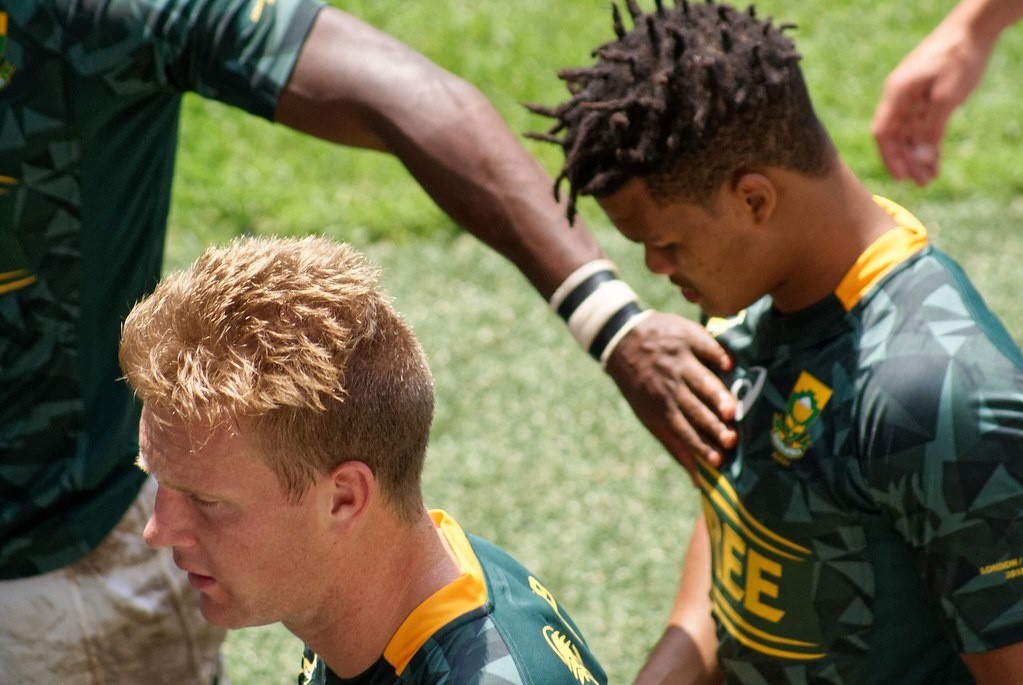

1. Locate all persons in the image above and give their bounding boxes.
[0,0,738,685]
[869,0,1023,186]
[520,1,1023,685]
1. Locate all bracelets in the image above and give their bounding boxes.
[549,258,656,372]
